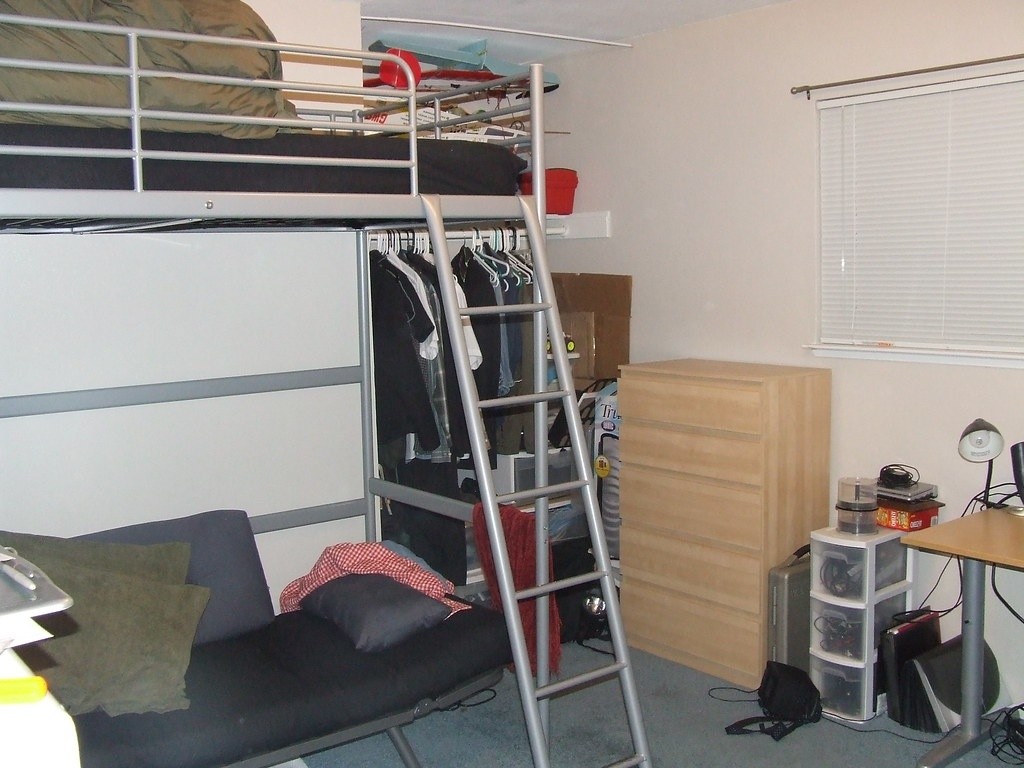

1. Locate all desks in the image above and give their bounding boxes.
[0,546,82,767]
[898,509,1023,768]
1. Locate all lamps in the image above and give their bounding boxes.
[957,418,1009,508]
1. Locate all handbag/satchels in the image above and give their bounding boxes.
[548,377,617,449]
[758,660,822,722]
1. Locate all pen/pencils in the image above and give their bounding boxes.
[5,560,34,579]
[1,564,35,590]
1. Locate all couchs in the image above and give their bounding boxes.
[1,497,513,767]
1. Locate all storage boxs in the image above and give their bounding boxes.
[878,507,937,532]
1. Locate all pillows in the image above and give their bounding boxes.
[0,529,192,587]
[70,509,278,644]
[23,558,212,715]
[310,570,449,652]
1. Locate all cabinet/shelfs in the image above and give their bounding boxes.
[805,524,913,720]
[614,360,832,692]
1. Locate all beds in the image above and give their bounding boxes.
[0,122,546,231]
